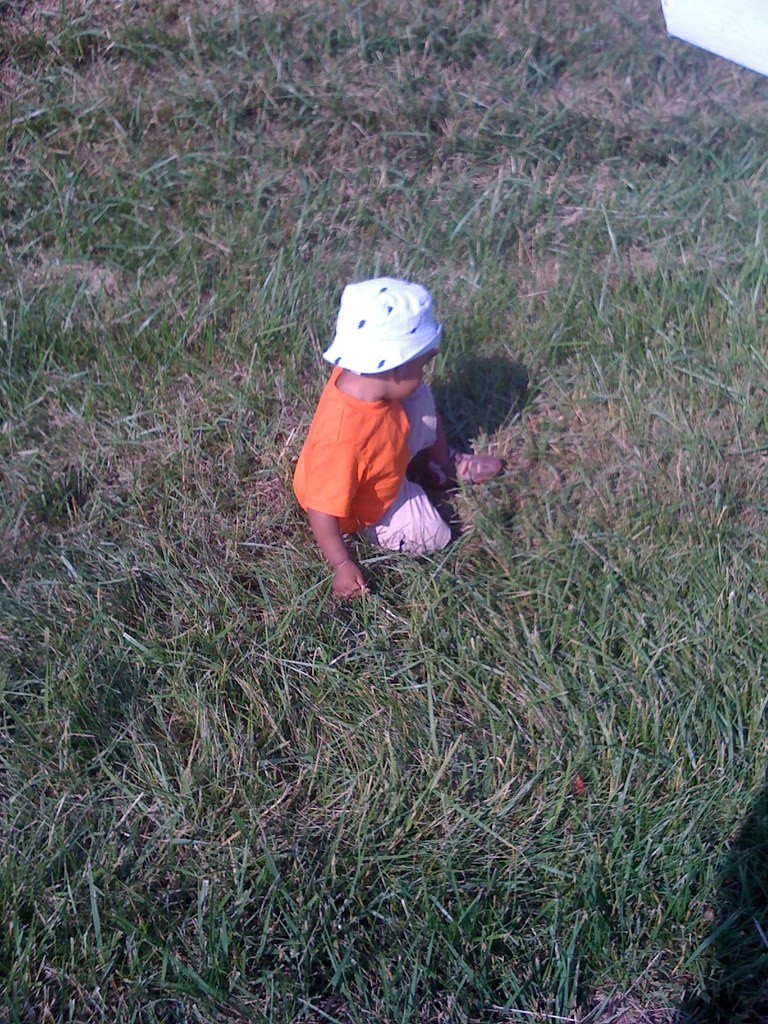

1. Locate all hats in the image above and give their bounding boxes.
[320,276,444,374]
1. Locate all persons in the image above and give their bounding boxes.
[292,276,504,599]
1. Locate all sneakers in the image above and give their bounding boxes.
[427,450,501,488]
